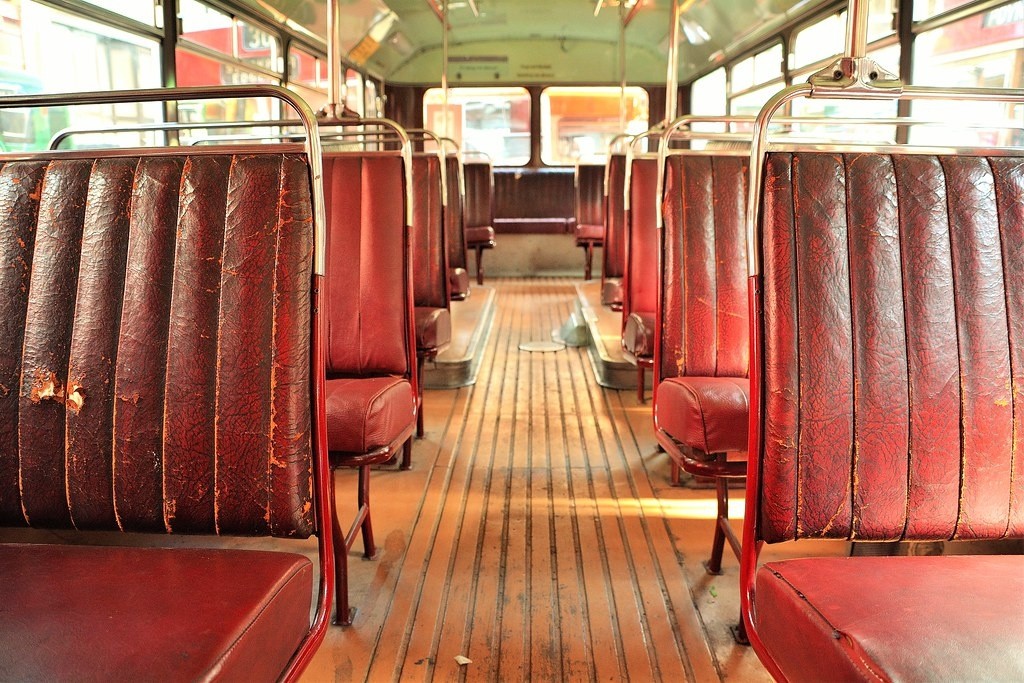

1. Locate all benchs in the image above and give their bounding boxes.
[0,82,1023,683]
